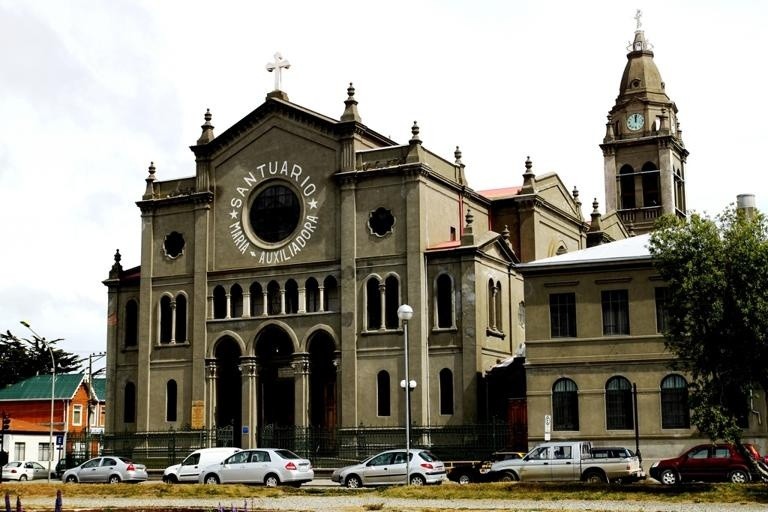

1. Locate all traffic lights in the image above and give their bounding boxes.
[3,413,11,429]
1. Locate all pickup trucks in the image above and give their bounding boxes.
[489,440,645,485]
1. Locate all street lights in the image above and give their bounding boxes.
[20,320,56,483]
[398,303,413,488]
[399,378,418,449]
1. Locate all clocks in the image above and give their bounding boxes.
[626,112,645,132]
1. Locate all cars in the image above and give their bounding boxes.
[591,446,646,485]
[61,454,149,484]
[339,448,446,488]
[2,460,55,481]
[198,447,314,487]
[447,451,527,484]
[650,443,760,486]
[56,458,90,481]
[163,446,243,484]
